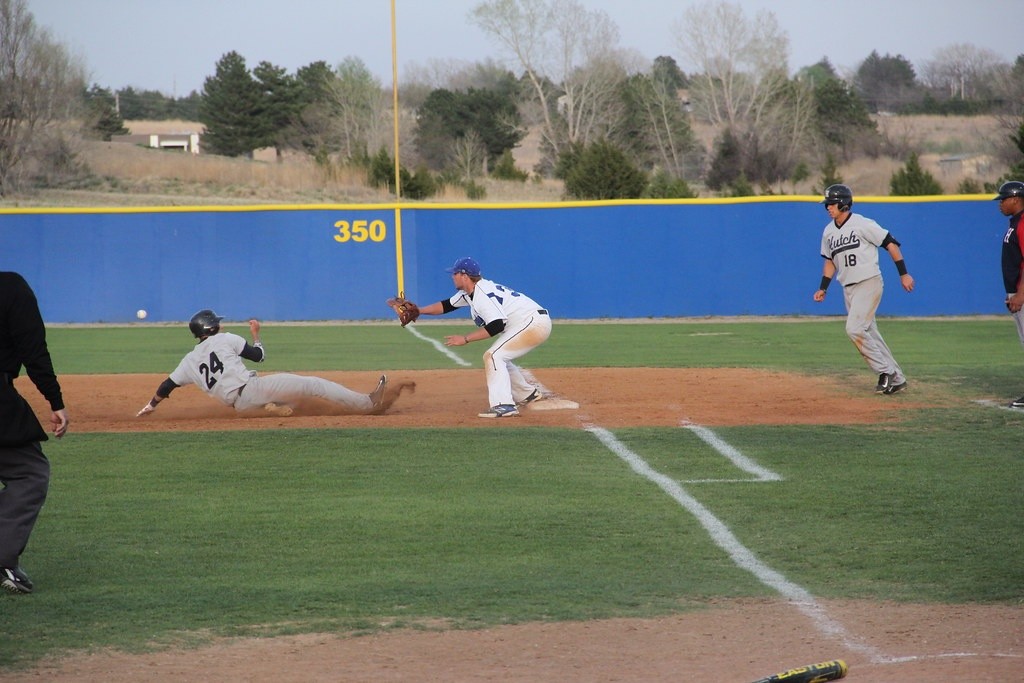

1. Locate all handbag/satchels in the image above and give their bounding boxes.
[0,384,49,447]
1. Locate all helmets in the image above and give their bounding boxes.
[819,184,852,211]
[994,181,1024,200]
[189,310,223,337]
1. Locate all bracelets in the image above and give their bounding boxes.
[150,398,159,407]
[465,336,468,343]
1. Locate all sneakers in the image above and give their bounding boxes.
[265,402,294,416]
[875,372,897,393]
[370,374,386,411]
[477,404,519,418]
[517,388,543,407]
[882,382,907,394]
[0,568,33,593]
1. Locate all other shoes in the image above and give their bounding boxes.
[1010,397,1024,409]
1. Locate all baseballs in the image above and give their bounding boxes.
[136,309,147,320]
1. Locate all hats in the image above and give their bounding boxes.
[445,257,481,276]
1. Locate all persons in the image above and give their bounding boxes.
[136,310,387,416]
[0,271,69,594]
[992,182,1024,408]
[386,257,552,418]
[813,184,916,393]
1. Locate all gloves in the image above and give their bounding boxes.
[136,401,155,417]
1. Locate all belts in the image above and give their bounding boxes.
[0,373,12,385]
[845,283,856,287]
[537,310,547,314]
[233,384,246,408]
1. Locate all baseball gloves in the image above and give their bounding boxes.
[385,296,420,327]
